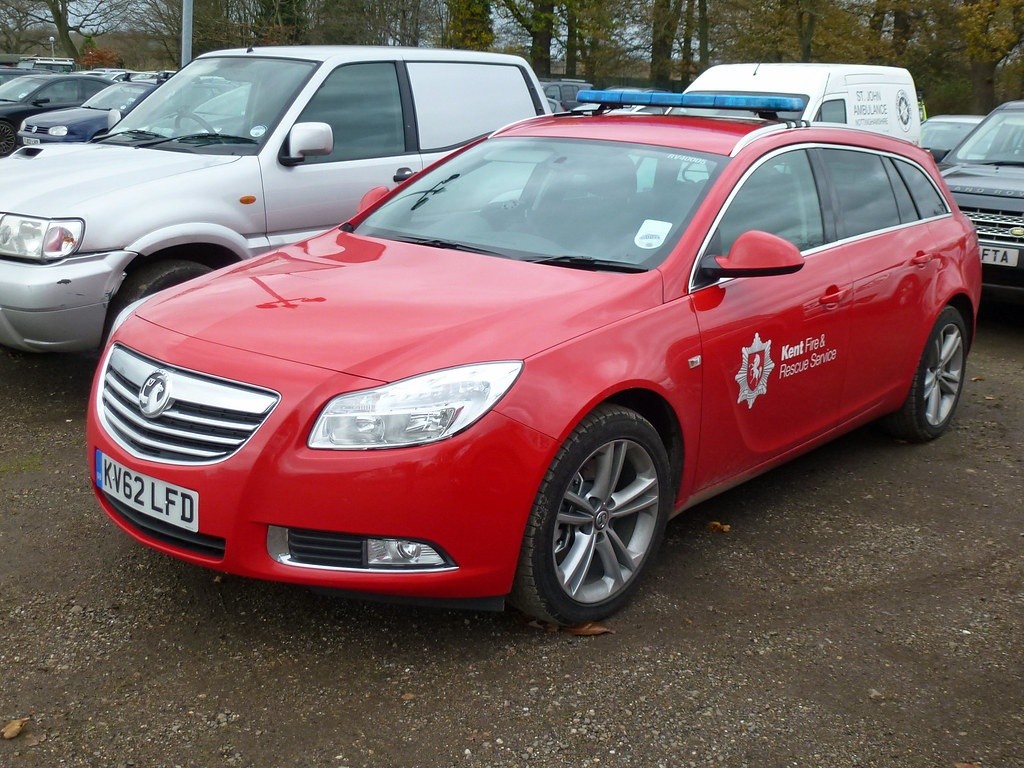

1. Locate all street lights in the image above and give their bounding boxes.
[49,36,56,61]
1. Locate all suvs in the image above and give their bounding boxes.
[600,86,675,115]
[18,60,75,73]
[920,100,1024,298]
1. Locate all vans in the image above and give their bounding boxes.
[538,77,594,110]
[0,49,555,358]
[633,64,921,192]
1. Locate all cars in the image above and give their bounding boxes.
[15,80,239,153]
[142,79,253,137]
[0,74,130,156]
[0,67,176,84]
[84,94,982,623]
[920,113,998,164]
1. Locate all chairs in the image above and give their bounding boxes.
[527,154,645,247]
